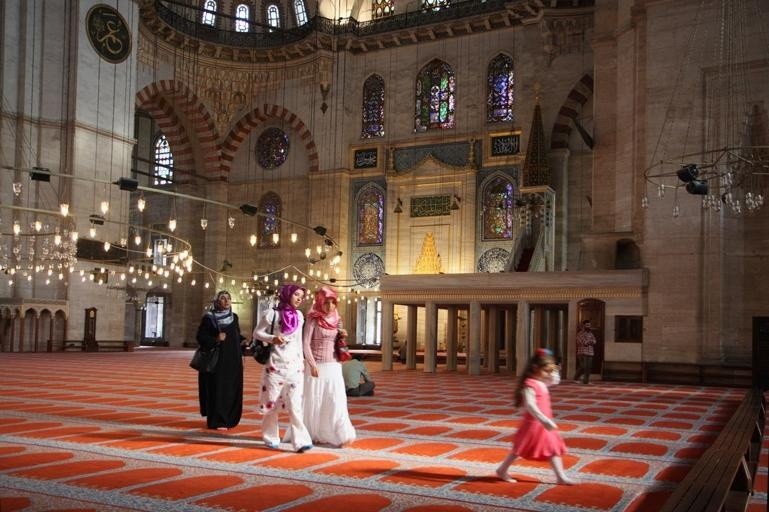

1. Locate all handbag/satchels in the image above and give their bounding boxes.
[251,337,273,364]
[336,337,352,362]
[190,346,220,373]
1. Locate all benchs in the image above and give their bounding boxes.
[660,392,768,511]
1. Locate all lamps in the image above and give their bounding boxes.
[640,0,768,219]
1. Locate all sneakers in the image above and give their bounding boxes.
[495,469,517,483]
[270,444,278,448]
[557,478,582,485]
[299,445,312,452]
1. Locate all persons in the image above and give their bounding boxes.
[496,347,579,484]
[280,285,356,448]
[194,290,252,430]
[573,320,596,385]
[251,284,312,452]
